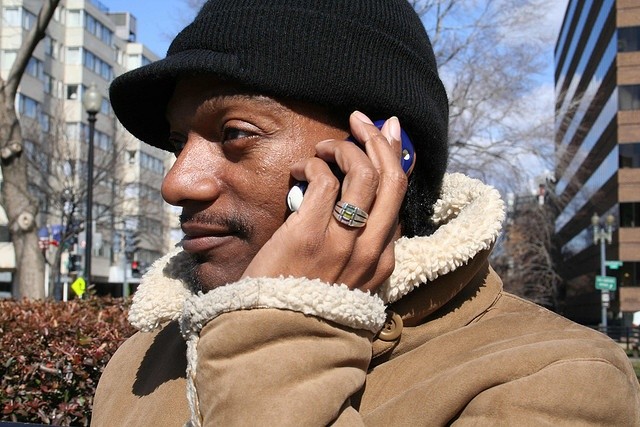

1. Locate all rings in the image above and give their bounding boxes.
[332,201,369,228]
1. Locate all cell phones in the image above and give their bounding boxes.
[287,117,416,219]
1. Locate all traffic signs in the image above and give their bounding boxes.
[595,275,618,291]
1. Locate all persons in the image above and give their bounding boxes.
[87,1,639,427]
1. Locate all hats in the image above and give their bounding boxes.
[109,0,448,179]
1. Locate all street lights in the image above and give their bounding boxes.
[590,213,616,336]
[79,78,104,300]
